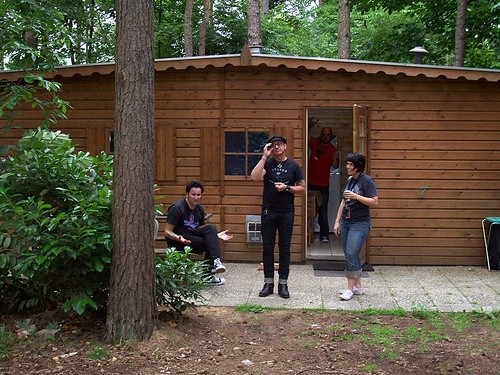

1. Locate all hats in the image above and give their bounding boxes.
[267,135,287,148]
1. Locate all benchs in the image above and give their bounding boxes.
[154,216,204,255]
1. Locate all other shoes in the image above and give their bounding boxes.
[321,235,329,243]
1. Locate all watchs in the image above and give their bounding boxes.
[285,186,290,192]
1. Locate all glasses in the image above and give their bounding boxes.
[271,143,284,148]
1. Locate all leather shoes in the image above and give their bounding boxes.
[278,282,289,297]
[259,283,274,297]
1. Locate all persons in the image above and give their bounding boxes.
[307,117,340,242]
[251,136,305,297]
[164,181,234,286]
[334,153,379,300]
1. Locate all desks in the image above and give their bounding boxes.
[307,190,324,246]
[482,216,500,271]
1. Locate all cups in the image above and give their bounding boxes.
[345,189,350,204]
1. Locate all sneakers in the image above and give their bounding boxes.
[210,258,226,273]
[201,277,225,286]
[340,289,353,300]
[340,287,364,295]
